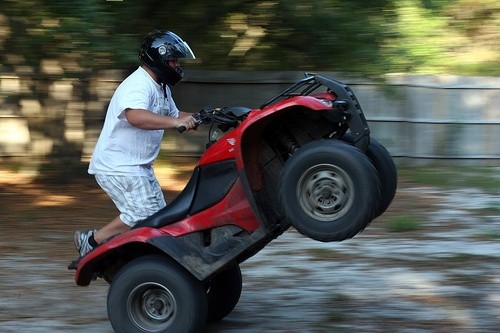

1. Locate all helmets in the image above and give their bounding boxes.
[139,29,196,86]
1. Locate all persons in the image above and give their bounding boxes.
[74,28,223,258]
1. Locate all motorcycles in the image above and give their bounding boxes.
[67,73,397,333]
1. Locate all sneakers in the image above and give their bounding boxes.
[74,229,95,258]
